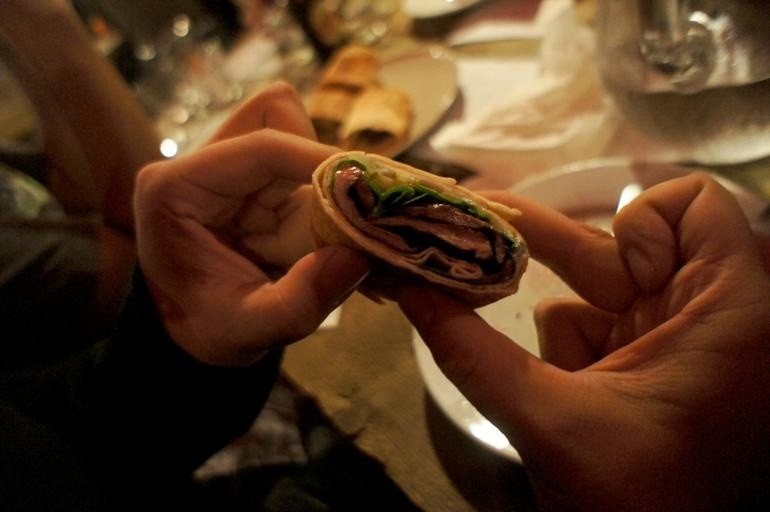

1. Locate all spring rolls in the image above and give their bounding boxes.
[311,151,530,309]
[308,46,412,145]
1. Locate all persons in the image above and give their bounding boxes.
[0,3,151,310]
[1,77,770,511]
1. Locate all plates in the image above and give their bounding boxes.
[370,45,453,158]
[410,156,770,467]
